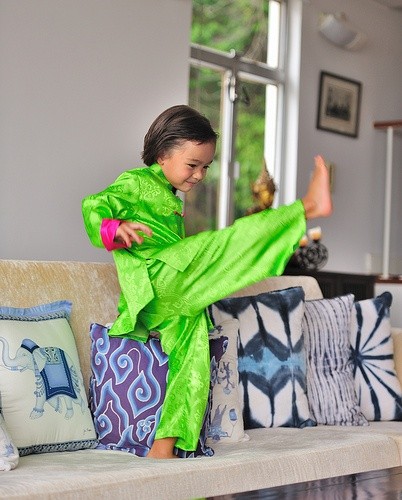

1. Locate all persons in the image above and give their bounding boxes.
[81,104,333,458]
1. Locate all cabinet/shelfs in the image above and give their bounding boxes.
[283,268,401,300]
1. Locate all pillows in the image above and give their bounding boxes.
[349,291,401,422]
[87,322,229,461]
[0,299,96,457]
[207,319,249,445]
[304,292,369,425]
[205,286,318,429]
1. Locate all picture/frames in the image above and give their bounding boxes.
[316,71,362,138]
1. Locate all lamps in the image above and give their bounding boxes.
[317,12,371,50]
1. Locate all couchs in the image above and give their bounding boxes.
[0,259,402,499]
